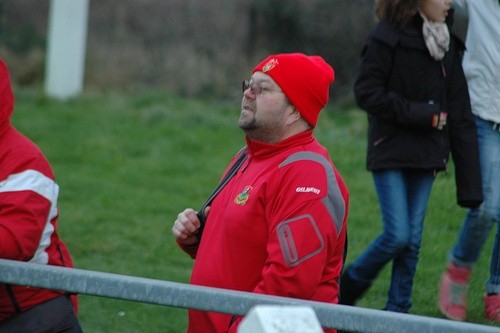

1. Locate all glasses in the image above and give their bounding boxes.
[241,79,283,95]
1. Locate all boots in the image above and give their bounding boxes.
[438,263,472,321]
[483,293,500,321]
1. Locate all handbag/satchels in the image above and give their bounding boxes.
[0,295,83,333]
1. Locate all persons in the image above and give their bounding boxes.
[172,53,348,333]
[0,59,77,323]
[339,0,484,313]
[439,0,500,321]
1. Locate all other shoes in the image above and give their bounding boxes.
[338,286,356,306]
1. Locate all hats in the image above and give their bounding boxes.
[252,52,335,128]
